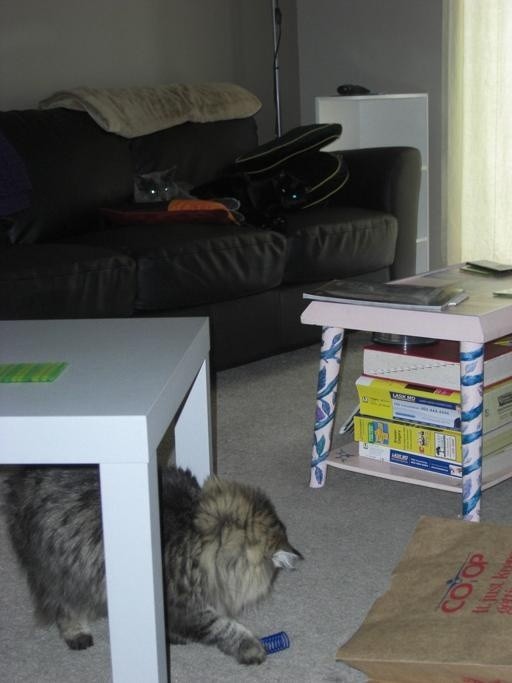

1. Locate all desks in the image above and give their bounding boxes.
[0,315,213,683]
[299,261,512,523]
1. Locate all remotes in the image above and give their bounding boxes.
[337,84,371,95]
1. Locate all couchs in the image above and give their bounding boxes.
[0,107,421,374]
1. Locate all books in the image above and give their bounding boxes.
[355,375,512,437]
[358,441,511,480]
[361,340,511,392]
[301,278,465,312]
[353,409,512,463]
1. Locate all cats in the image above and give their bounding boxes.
[133,163,245,226]
[4,465,307,669]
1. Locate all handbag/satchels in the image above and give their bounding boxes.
[237,122,349,209]
